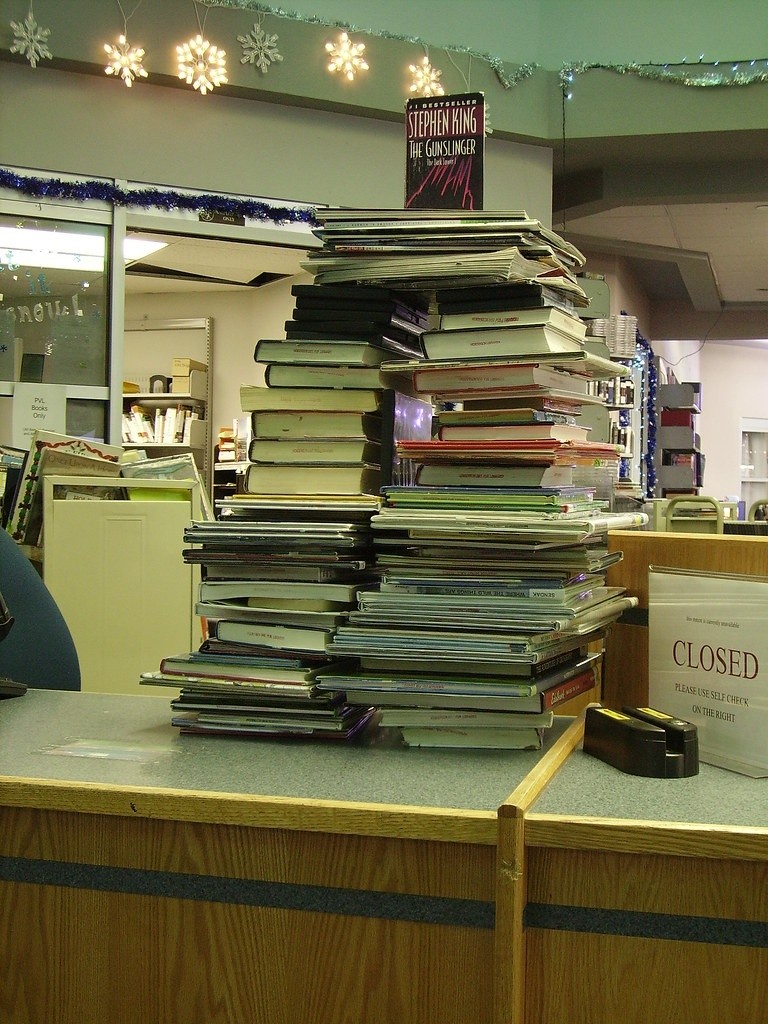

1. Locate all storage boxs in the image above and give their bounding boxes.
[170,358,207,393]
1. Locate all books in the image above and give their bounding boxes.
[585,376,644,497]
[122,403,204,443]
[660,381,704,487]
[404,91,486,208]
[0,430,216,548]
[676,509,718,518]
[138,206,649,750]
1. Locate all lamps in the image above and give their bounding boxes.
[325,32,370,81]
[236,12,283,73]
[408,44,444,97]
[175,0,228,95]
[465,54,493,138]
[104,0,148,88]
[9,0,53,68]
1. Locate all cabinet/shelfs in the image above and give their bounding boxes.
[17,472,201,696]
[211,443,258,521]
[122,370,208,485]
[604,352,707,500]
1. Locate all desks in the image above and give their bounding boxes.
[0,688,768,1023]
